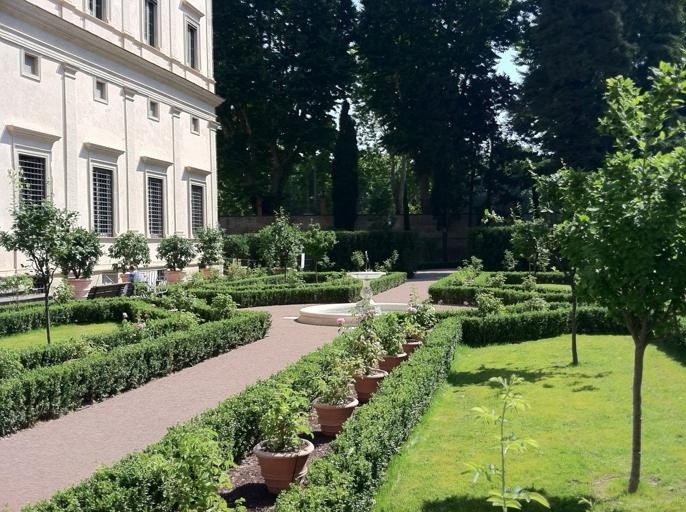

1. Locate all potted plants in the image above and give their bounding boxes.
[254,384,313,491]
[315,294,436,436]
[109,227,242,285]
[58,228,106,300]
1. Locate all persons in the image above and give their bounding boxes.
[127,265,142,283]
